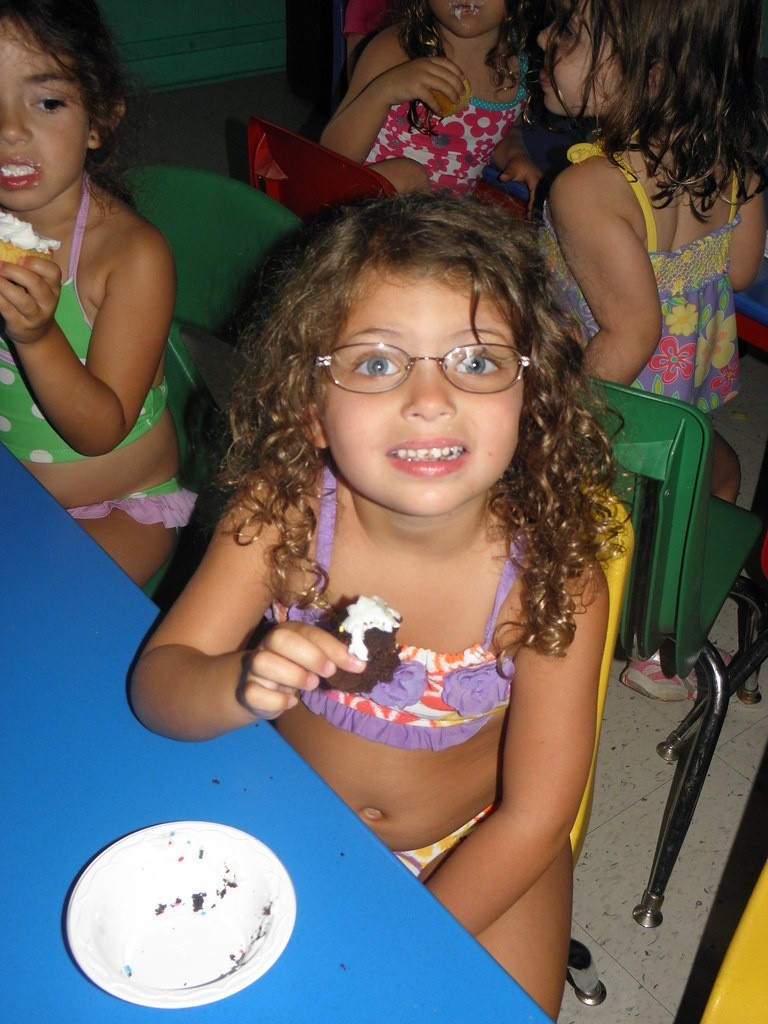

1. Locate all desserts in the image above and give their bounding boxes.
[0,213,62,264]
[314,594,403,694]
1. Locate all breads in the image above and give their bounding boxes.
[427,74,472,117]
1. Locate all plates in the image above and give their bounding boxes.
[66,820,298,1010]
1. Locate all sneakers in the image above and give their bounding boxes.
[620,647,733,703]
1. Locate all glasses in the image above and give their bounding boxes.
[313,343,531,394]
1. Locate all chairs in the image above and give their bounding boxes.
[248,115,403,232]
[328,0,349,116]
[110,162,309,617]
[573,372,768,931]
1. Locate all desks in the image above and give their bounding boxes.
[0,444,558,1024]
[479,167,768,366]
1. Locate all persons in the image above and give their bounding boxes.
[130,188,632,1024]
[0,0,202,585]
[320,0,543,222]
[341,0,401,85]
[534,0,768,702]
